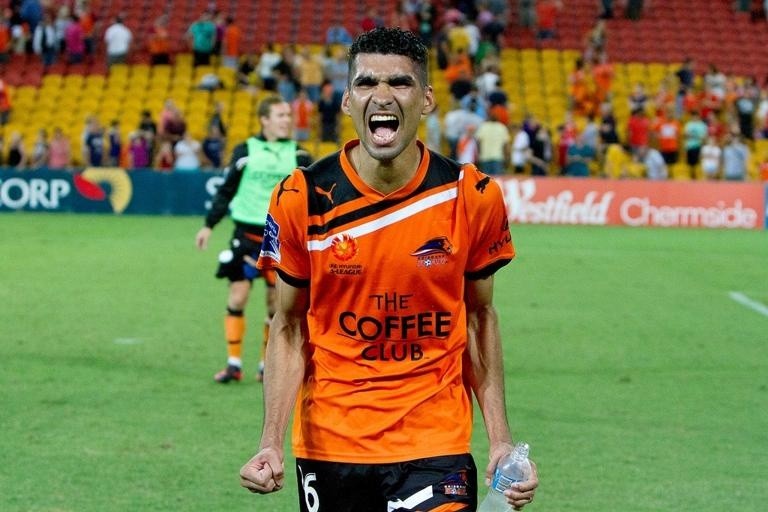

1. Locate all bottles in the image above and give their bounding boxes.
[476,440,532,511]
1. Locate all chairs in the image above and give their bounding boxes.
[0,0,768,182]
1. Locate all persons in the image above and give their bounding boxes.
[198,98,315,384]
[8,126,73,168]
[238,0,767,182]
[202,73,226,90]
[81,97,228,171]
[0,79,12,123]
[0,0,233,71]
[236,25,540,511]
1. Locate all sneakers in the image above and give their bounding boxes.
[214,365,243,384]
